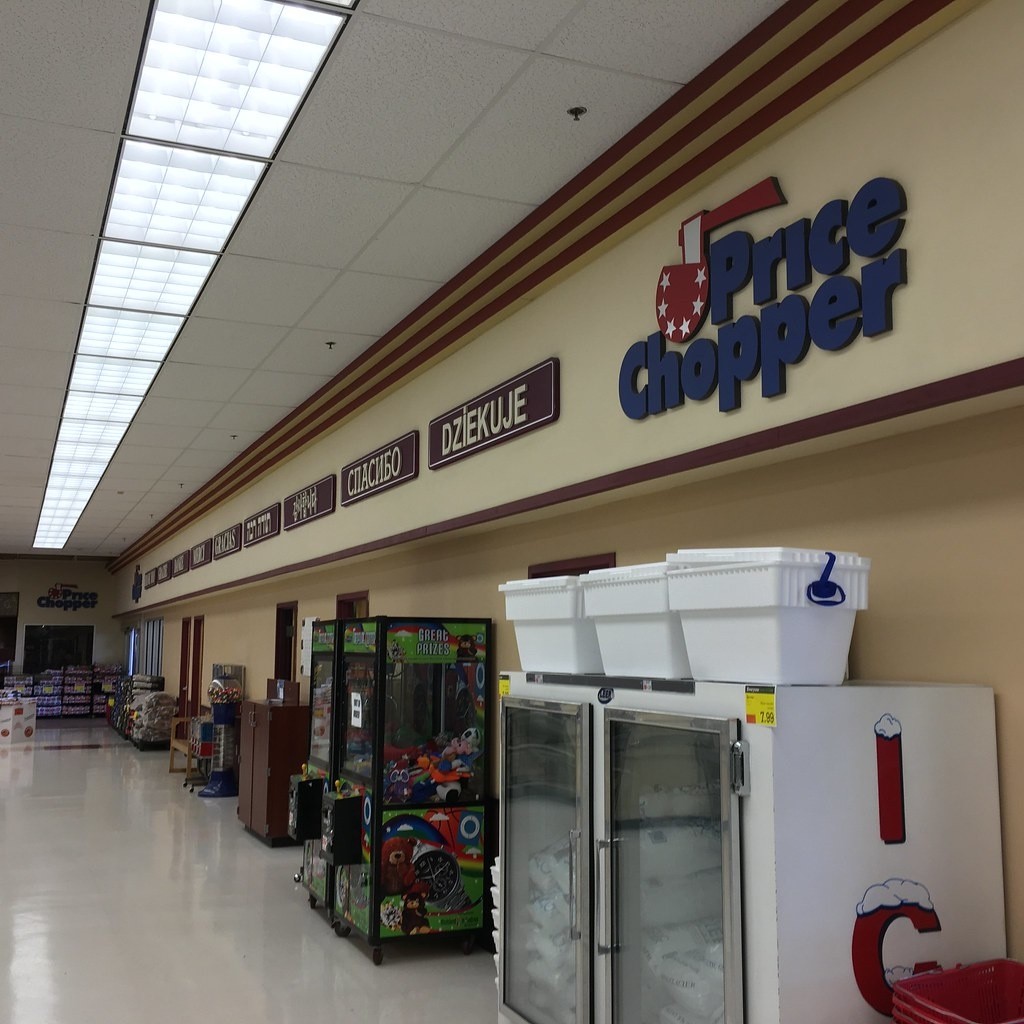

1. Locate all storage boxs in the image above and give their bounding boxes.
[498,547,871,685]
[892,958,1024,1024]
[4,664,122,716]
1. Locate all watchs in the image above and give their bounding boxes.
[410,838,472,913]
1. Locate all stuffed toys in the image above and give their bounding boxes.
[379,898,402,930]
[403,893,430,936]
[380,837,429,902]
[347,727,484,804]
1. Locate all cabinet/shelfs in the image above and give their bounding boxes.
[7,659,122,719]
[169,717,206,778]
[238,698,309,847]
